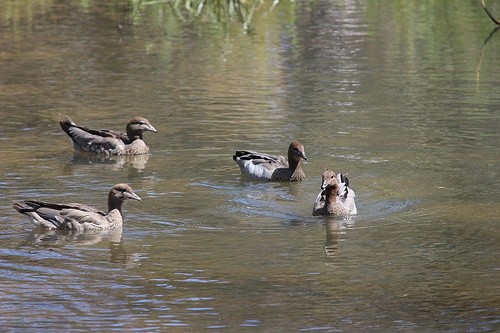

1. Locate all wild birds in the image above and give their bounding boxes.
[233,142,309,183]
[312,169,357,217]
[58,115,157,157]
[11,183,142,235]
